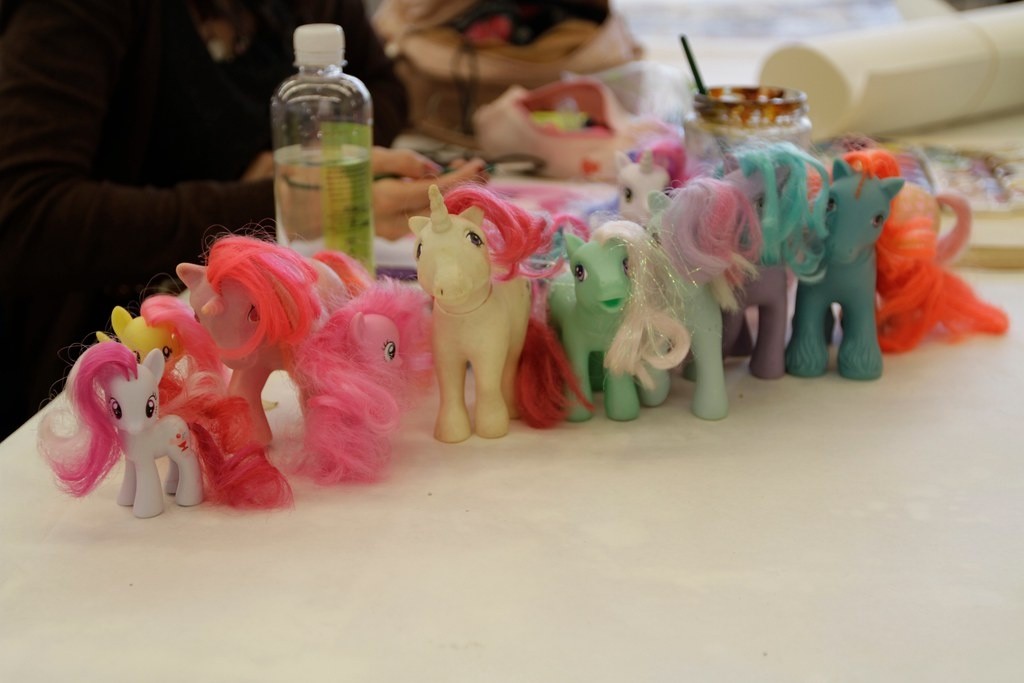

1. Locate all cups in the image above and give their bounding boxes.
[683,82,811,177]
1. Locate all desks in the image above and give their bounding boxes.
[0,40,1023,683]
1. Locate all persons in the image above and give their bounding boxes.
[0,0,491,446]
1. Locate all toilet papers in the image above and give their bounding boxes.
[756,1,1024,154]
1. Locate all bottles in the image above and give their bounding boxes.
[271,24,376,282]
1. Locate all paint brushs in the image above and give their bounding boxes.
[680,34,707,94]
